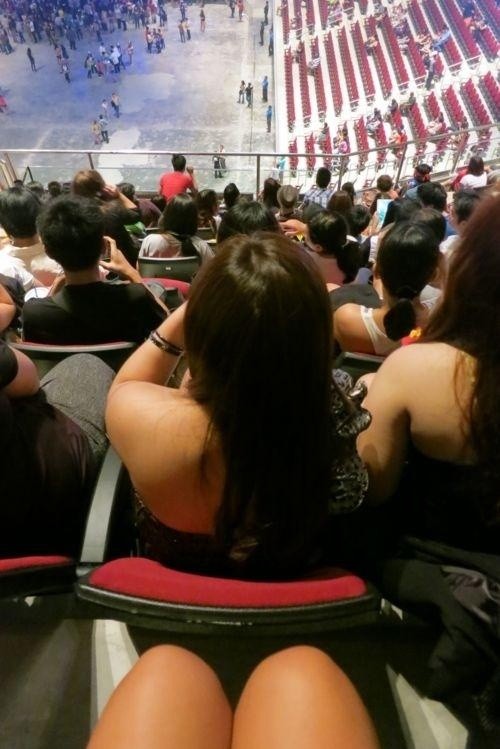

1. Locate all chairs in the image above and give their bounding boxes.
[280,0,500,178]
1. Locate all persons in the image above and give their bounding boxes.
[0,3,500,749]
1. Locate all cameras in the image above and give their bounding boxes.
[99,240,111,260]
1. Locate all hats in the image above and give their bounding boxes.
[277,184,299,208]
